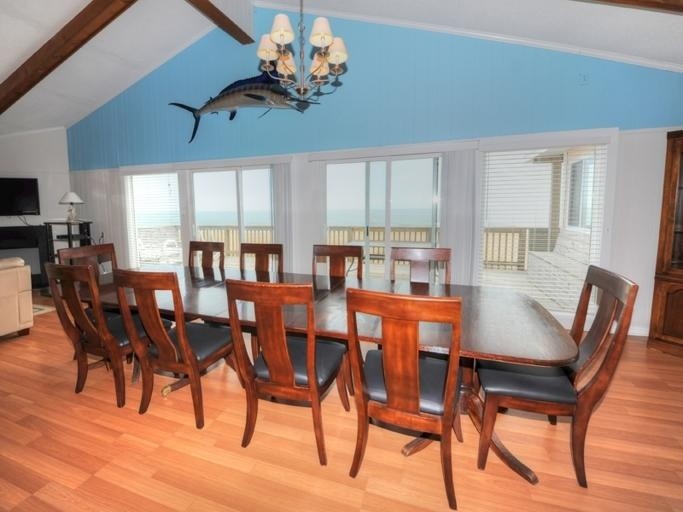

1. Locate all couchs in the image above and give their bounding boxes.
[0,254,32,340]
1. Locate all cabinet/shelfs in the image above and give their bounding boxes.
[647,130,683,358]
[47,221,91,262]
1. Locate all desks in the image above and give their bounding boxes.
[75,268,580,484]
[346,240,435,277]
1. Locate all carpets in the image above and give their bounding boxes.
[32,303,53,319]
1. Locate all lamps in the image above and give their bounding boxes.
[255,1,348,101]
[59,191,82,221]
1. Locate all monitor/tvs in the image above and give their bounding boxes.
[0,177,40,216]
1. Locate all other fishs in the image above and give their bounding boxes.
[168,71,320,144]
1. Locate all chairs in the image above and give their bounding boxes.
[223,278,349,464]
[56,243,114,362]
[343,287,462,509]
[185,238,225,268]
[43,263,171,408]
[309,243,362,276]
[113,268,244,429]
[476,265,639,487]
[387,245,451,285]
[237,242,282,269]
